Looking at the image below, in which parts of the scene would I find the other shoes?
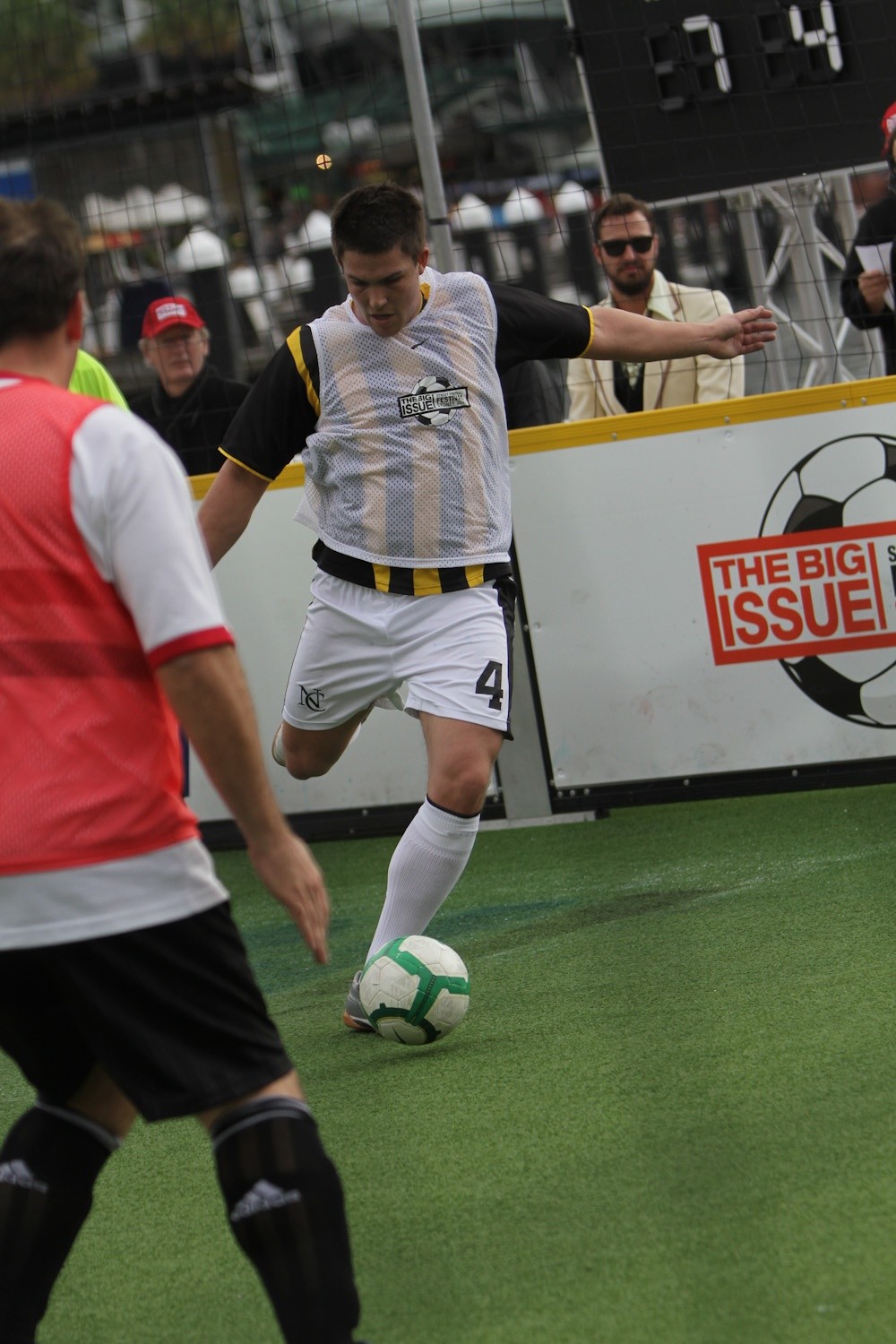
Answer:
[272,723,287,767]
[344,971,376,1031]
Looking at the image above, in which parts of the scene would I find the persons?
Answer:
[0,193,365,1343]
[839,100,896,381]
[562,194,744,424]
[199,186,782,1030]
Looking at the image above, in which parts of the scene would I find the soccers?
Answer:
[358,934,470,1046]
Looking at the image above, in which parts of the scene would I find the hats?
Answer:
[142,297,204,338]
[880,102,896,154]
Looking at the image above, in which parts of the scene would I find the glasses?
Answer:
[602,236,654,257]
